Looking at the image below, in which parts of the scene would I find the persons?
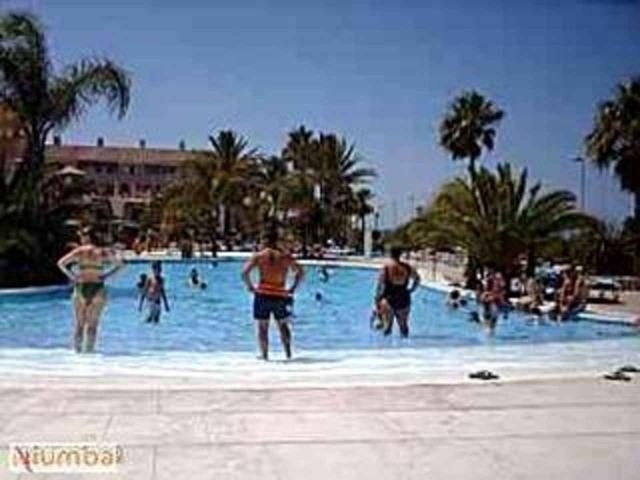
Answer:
[371,245,420,337]
[137,260,170,323]
[312,265,328,304]
[56,224,121,352]
[188,260,218,292]
[240,228,303,360]
[425,244,438,281]
[445,245,591,334]
